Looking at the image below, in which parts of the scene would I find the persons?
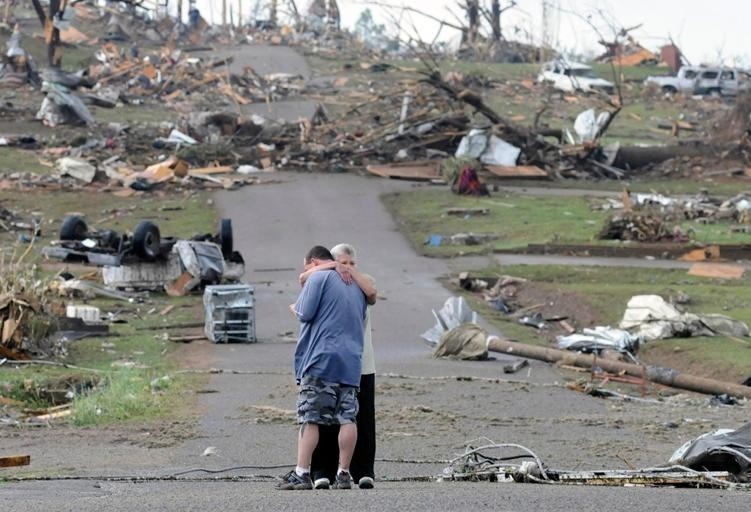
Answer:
[274,243,378,489]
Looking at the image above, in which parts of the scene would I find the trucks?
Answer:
[44,217,246,296]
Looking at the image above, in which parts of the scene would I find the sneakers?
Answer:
[274,470,375,490]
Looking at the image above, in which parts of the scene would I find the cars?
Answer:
[643,65,751,97]
[537,60,614,97]
